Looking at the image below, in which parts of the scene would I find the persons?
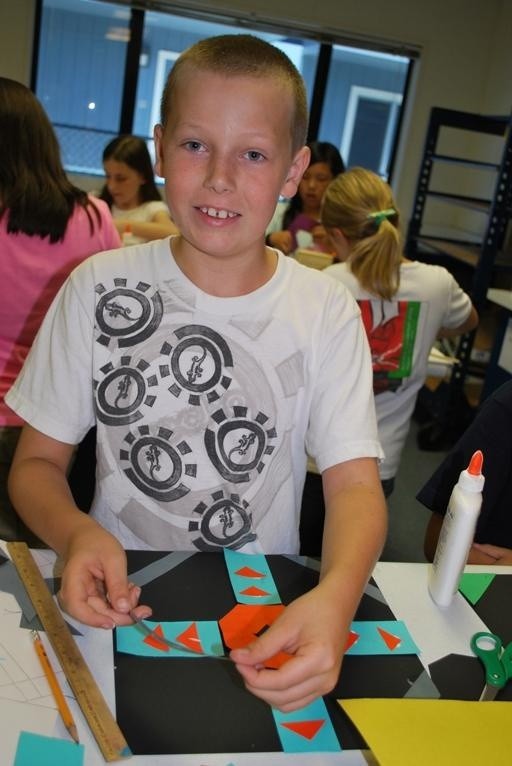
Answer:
[89,133,183,248]
[0,76,124,551]
[262,138,351,266]
[415,378,511,568]
[299,164,481,557]
[2,33,390,716]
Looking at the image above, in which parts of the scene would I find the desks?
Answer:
[0,540,512,766]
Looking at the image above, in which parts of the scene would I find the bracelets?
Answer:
[263,233,274,248]
[124,218,133,234]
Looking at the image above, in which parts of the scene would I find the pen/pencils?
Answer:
[31,628,79,745]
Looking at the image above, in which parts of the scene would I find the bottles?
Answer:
[430,448,487,608]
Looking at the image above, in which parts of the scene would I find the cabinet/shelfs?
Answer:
[398,106,512,451]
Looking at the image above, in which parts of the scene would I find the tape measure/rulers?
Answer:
[7,541,132,763]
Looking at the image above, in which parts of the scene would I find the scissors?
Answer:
[471,632,511,701]
